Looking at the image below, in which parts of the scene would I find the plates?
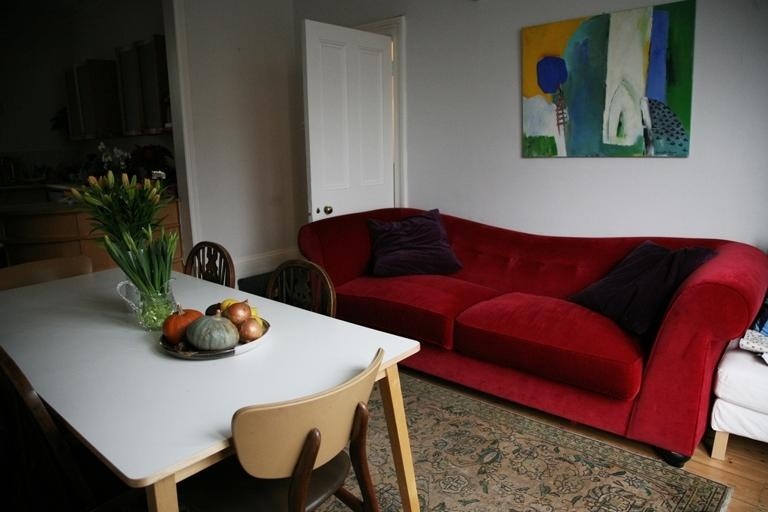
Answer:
[157,318,271,361]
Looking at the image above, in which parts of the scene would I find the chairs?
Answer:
[263,254,336,319]
[178,238,241,290]
[229,344,387,511]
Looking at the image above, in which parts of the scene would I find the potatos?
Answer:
[229,300,253,322]
[242,319,263,341]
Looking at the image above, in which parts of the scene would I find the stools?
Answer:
[705,334,768,463]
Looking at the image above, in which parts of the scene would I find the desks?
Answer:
[0,260,427,511]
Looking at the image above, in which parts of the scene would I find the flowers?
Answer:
[53,164,176,242]
[102,225,184,296]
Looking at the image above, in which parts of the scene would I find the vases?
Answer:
[131,282,187,330]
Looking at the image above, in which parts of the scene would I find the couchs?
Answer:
[294,200,768,468]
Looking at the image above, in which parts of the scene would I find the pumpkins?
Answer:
[159,312,202,345]
[183,308,242,353]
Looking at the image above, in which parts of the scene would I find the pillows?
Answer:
[564,238,718,360]
[362,205,465,281]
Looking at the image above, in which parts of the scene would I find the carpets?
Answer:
[301,358,735,511]
